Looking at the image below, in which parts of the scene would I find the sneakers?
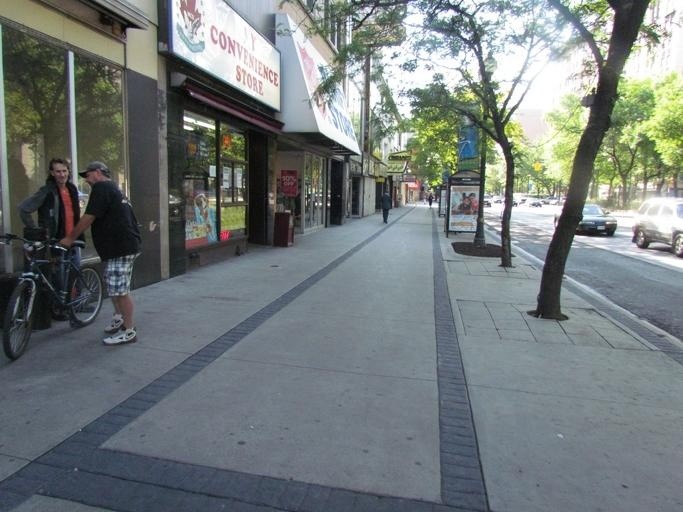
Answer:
[72,301,95,313]
[52,311,69,322]
[101,327,137,347]
[104,312,123,333]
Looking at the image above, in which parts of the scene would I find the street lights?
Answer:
[471,50,498,250]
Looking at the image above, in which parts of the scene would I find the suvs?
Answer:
[627,195,682,257]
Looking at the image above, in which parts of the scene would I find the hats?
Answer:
[78,161,110,179]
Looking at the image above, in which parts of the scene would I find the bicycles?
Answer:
[0,231,103,361]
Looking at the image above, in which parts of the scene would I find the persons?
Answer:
[380,192,391,225]
[17,157,97,322]
[427,192,434,209]
[57,159,145,346]
[452,192,478,215]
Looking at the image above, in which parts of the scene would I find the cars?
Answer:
[481,191,568,210]
[169,193,183,220]
[552,203,617,237]
[72,189,91,210]
